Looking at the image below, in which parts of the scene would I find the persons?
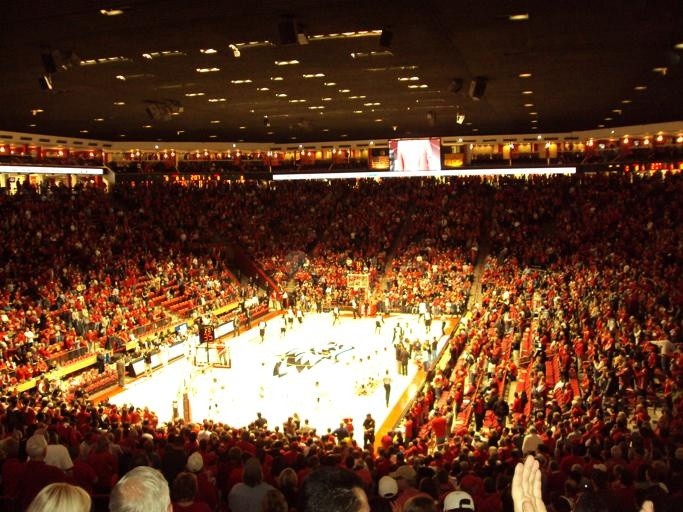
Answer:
[1,153,683,510]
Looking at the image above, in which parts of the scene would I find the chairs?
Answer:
[131,271,193,317]
[452,335,573,436]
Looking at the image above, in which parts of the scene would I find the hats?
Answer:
[279,468,298,487]
[378,476,398,499]
[442,491,474,512]
[593,464,607,472]
[389,466,416,481]
[187,452,203,473]
